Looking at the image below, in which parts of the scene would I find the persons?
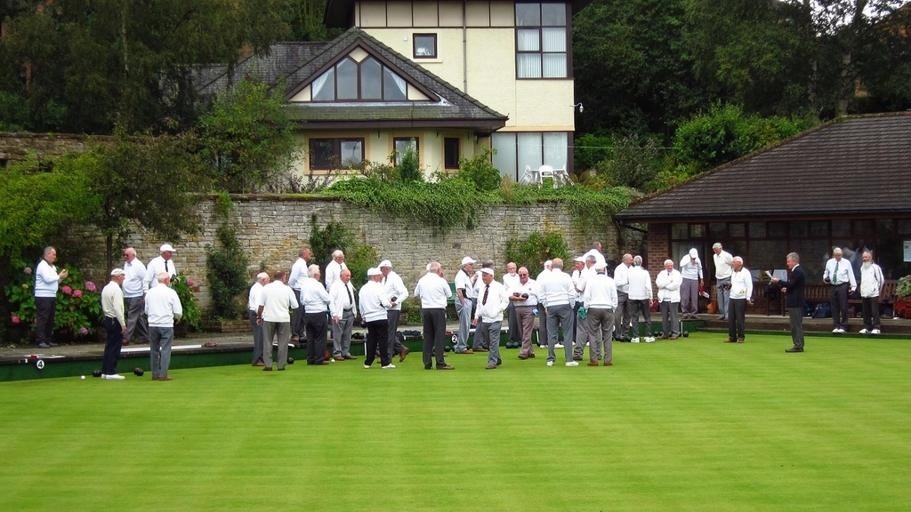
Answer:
[614,254,654,343]
[823,247,857,332]
[656,258,683,340]
[358,260,410,370]
[454,256,618,370]
[712,243,733,321]
[250,271,298,370]
[288,247,357,364]
[583,242,606,266]
[33,245,69,348]
[724,256,754,343]
[769,248,810,355]
[414,261,454,371]
[858,252,887,335]
[679,248,704,320]
[100,242,183,381]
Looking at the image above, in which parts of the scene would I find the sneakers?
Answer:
[832,328,847,334]
[299,337,307,343]
[363,345,409,368]
[102,373,125,380]
[859,328,880,334]
[456,340,612,369]
[736,337,744,343]
[631,333,678,343]
[724,337,737,342]
[291,335,300,342]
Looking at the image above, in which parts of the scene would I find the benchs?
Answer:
[748,279,897,318]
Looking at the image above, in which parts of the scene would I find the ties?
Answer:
[345,284,352,304]
[165,261,168,272]
[833,262,838,283]
[482,284,490,305]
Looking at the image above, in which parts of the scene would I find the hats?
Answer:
[574,257,585,265]
[689,248,699,259]
[461,256,476,265]
[160,244,176,252]
[367,267,382,275]
[379,260,393,268]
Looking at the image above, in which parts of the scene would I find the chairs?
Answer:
[519,165,569,189]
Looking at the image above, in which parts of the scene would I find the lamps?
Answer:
[570,102,583,112]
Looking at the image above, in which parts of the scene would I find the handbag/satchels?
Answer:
[812,303,830,318]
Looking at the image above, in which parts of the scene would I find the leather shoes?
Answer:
[785,347,803,352]
[437,364,453,369]
[691,316,701,319]
[681,316,687,319]
[39,342,57,348]
[307,352,357,364]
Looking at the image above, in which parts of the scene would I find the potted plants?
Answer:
[896,276,911,321]
